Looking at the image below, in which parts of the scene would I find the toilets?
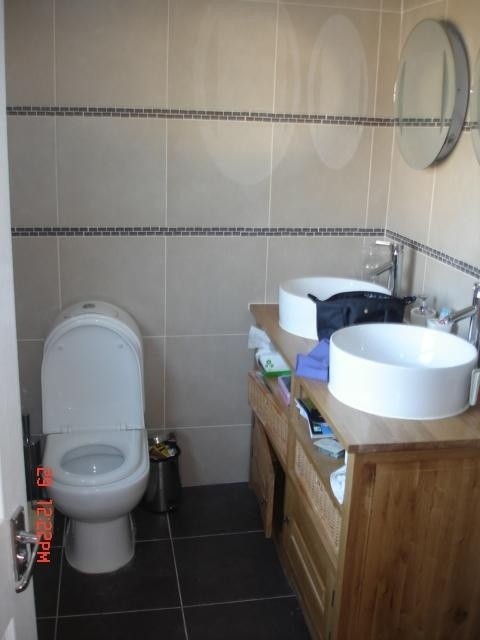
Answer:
[40,299,150,575]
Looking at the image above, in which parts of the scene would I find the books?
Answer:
[294,397,345,459]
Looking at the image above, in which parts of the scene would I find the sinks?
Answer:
[328,322,477,420]
[279,276,392,340]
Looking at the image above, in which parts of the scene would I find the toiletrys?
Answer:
[411,296,435,325]
[427,307,453,332]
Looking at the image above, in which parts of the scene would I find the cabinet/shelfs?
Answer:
[247,303,479,638]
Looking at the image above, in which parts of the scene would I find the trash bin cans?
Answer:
[148,441,182,511]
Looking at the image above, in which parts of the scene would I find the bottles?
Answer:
[364,243,383,285]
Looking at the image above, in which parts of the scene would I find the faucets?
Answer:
[443,280,479,342]
[369,242,398,298]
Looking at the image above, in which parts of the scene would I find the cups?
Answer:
[411,306,453,334]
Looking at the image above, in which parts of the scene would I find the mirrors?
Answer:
[390,18,471,169]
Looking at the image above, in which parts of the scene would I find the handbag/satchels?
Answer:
[308,290,416,342]
